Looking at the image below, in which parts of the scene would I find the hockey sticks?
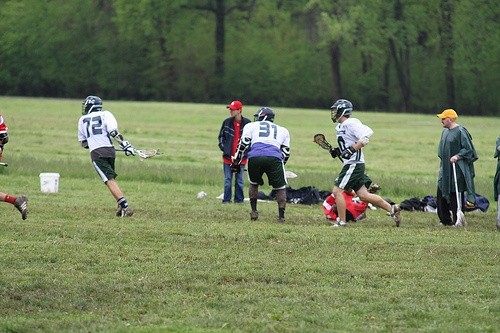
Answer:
[114,149,161,158]
[0,163,8,167]
[452,162,468,228]
[230,167,297,179]
[314,133,343,162]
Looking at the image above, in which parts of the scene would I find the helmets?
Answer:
[82,95,103,115]
[253,107,275,123]
[330,99,352,123]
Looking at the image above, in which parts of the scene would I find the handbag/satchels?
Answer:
[287,185,319,205]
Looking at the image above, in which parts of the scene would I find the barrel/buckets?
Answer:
[39,172,61,193]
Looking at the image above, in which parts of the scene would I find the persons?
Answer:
[230,107,291,222]
[218,100,252,204]
[330,99,401,227]
[77,96,138,219]
[0,116,29,220]
[435,109,478,227]
[494,134,500,231]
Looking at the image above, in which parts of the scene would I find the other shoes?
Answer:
[277,217,285,222]
[251,212,258,220]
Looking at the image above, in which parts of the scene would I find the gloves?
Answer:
[230,157,241,173]
[119,140,136,156]
[342,146,356,160]
[330,147,341,158]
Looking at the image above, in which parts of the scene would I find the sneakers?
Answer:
[387,205,400,227]
[330,217,347,227]
[14,196,29,220]
[121,207,133,217]
[116,205,122,216]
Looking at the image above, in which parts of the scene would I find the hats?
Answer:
[436,109,458,119]
[226,101,242,110]
